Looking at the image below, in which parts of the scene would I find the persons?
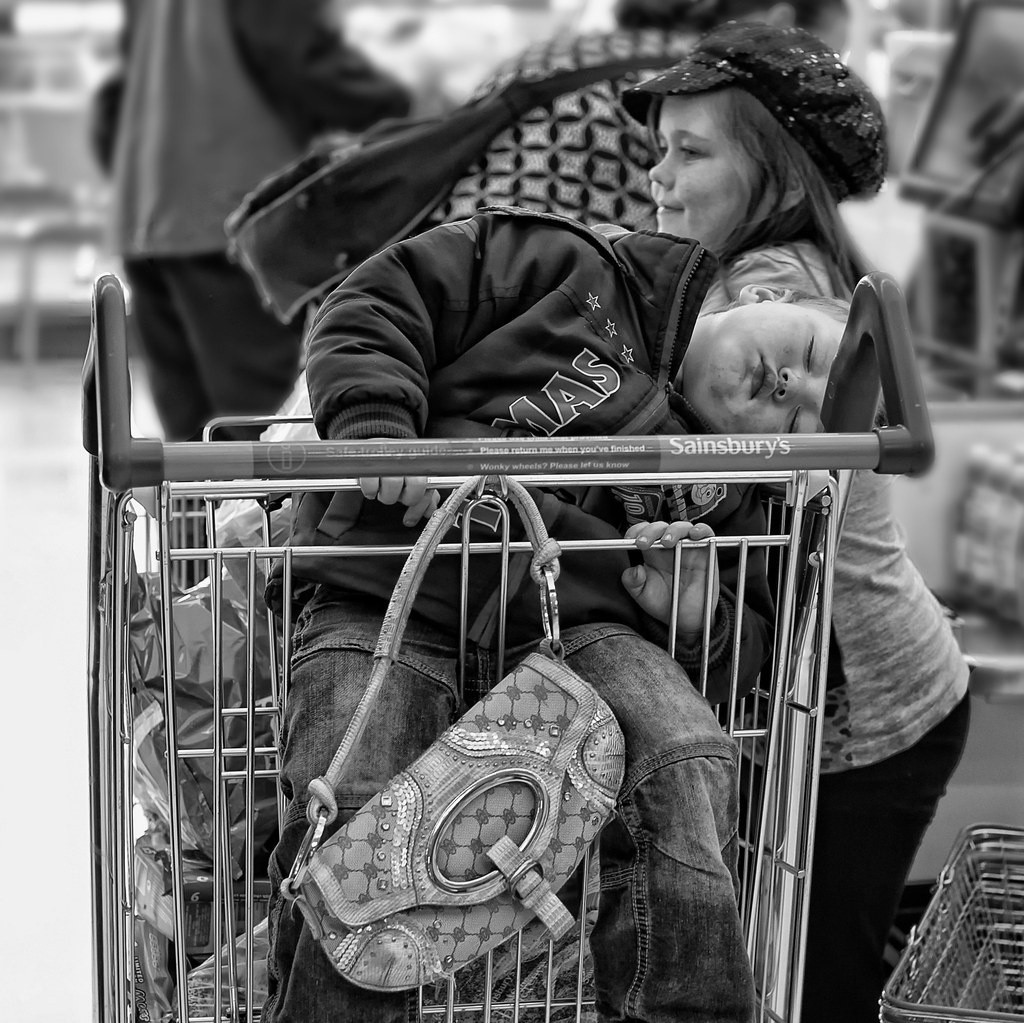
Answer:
[264,0,974,1023]
[88,0,449,583]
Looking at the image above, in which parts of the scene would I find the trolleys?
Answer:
[81,269,936,1022]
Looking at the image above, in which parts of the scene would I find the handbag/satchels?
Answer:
[221,55,684,326]
[279,475,625,992]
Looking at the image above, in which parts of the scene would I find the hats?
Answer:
[620,21,888,203]
